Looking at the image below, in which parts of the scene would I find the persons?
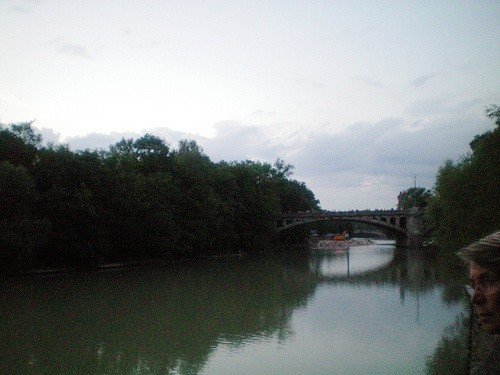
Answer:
[457,232,500,375]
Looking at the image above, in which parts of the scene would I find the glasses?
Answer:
[466,270,500,298]
